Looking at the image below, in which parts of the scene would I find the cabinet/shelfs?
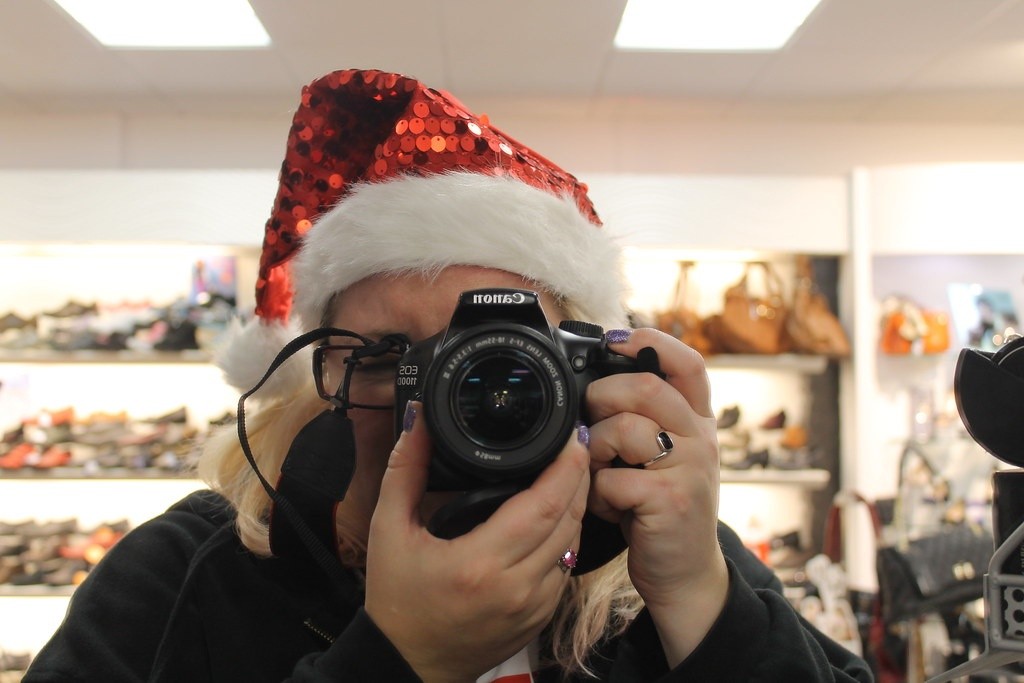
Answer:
[0,349,830,683]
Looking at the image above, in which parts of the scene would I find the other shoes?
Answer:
[714,407,818,569]
[0,292,236,669]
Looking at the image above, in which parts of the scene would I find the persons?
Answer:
[15,67,876,683]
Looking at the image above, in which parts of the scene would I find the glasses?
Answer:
[312,343,401,410]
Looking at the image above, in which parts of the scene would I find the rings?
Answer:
[557,548,578,575]
[639,428,676,469]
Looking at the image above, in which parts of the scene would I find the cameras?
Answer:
[392,287,667,579]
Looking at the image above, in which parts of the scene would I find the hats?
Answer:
[215,67,630,396]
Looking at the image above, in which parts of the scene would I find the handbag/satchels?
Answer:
[875,443,995,624]
[877,300,950,355]
[804,488,878,656]
[658,259,852,358]
